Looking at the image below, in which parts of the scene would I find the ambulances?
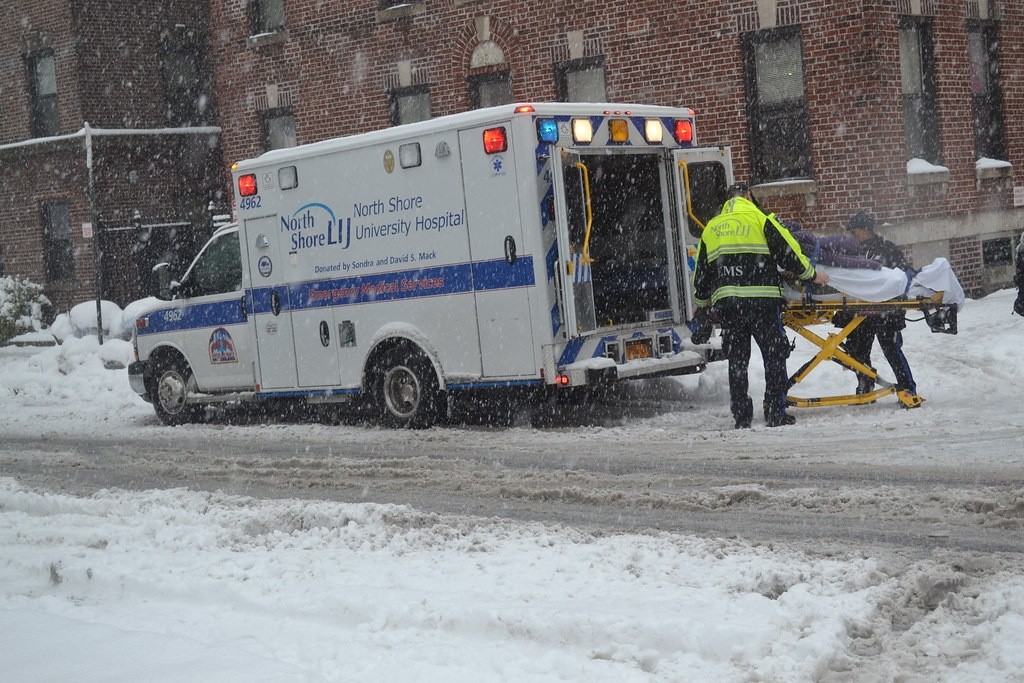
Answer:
[126,101,737,429]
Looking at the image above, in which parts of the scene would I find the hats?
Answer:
[727,183,759,207]
[847,211,873,230]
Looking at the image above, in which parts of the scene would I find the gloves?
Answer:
[694,307,707,325]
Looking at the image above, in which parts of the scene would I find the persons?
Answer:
[846,211,917,405]
[693,183,829,430]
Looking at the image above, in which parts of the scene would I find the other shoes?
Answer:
[901,388,921,409]
[856,384,872,394]
[735,414,752,429]
[766,412,795,427]
